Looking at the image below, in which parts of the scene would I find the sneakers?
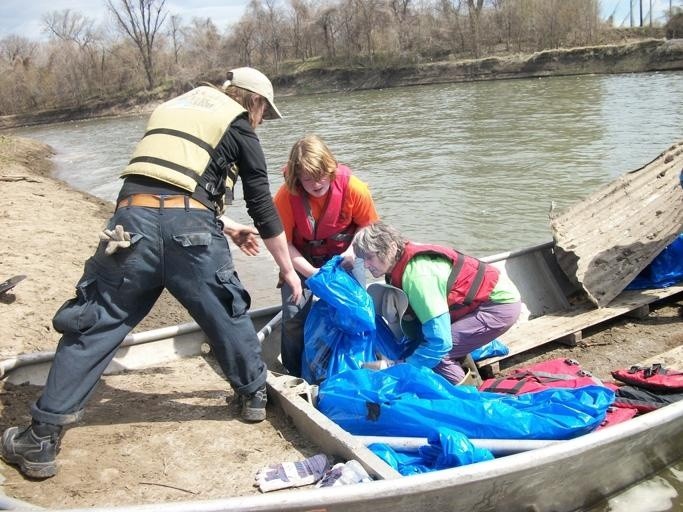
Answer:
[235,381,268,421]
[2,421,64,479]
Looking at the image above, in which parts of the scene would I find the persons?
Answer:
[0,66,301,481]
[272,137,380,378]
[350,223,523,386]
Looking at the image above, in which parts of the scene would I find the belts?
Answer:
[116,194,209,210]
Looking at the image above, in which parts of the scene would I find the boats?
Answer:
[0,144,681,507]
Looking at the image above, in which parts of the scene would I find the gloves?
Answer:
[254,454,329,493]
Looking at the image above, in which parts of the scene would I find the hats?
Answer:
[226,67,282,121]
